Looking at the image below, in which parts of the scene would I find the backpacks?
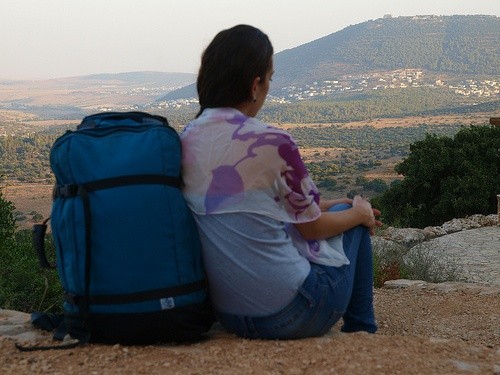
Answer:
[14,112,213,351]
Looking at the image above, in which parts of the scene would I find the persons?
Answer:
[178,25,383,341]
